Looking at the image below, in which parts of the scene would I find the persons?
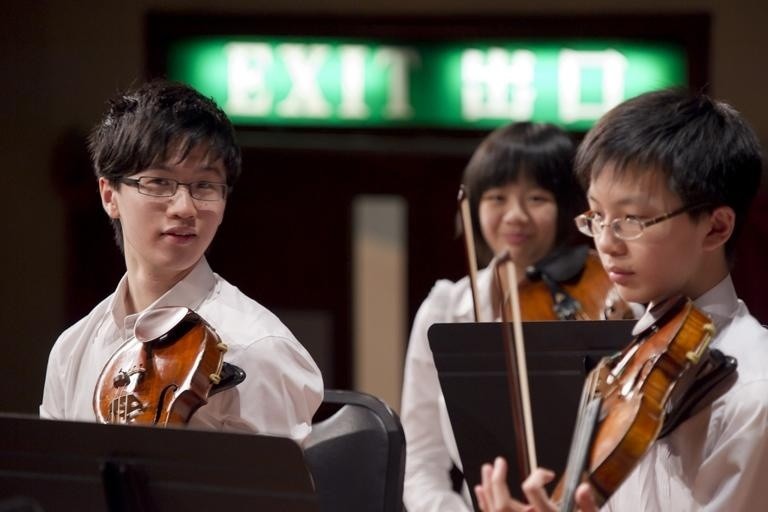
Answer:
[473,89,768,512]
[39,77,323,444]
[401,123,644,512]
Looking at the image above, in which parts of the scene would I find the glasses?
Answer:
[120,174,233,202]
[573,206,690,242]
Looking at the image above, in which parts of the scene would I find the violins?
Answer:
[551,296,739,510]
[505,249,618,323]
[93,309,245,423]
